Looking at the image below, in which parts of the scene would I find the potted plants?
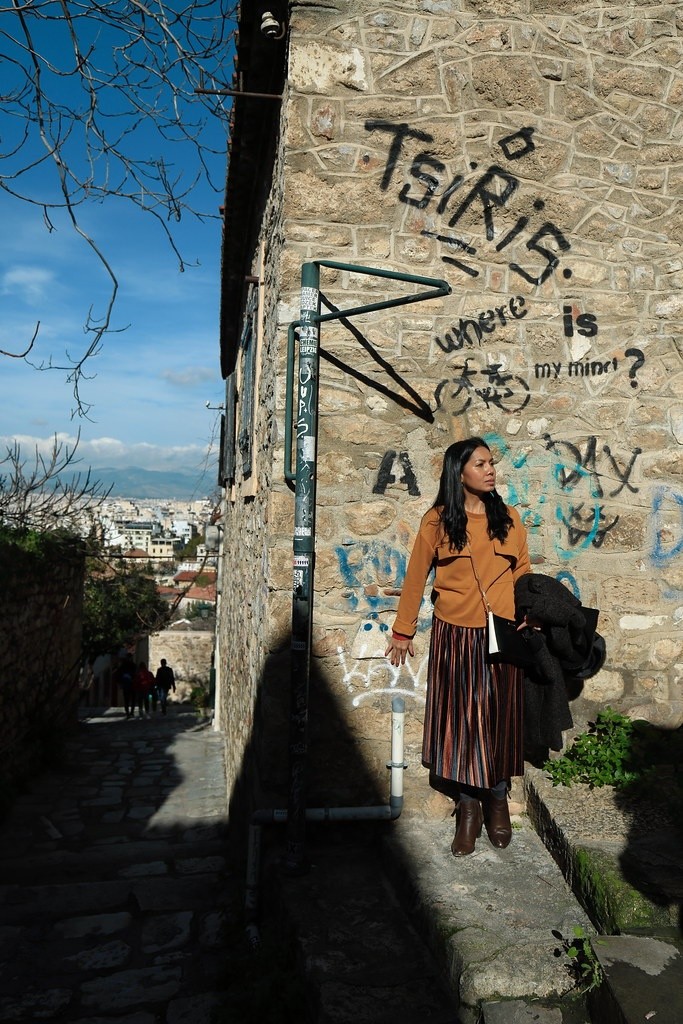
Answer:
[192,695,211,717]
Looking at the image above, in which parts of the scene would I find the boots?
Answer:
[451,790,512,857]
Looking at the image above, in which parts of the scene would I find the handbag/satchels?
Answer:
[487,611,527,667]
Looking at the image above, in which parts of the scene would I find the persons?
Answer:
[384,435,545,858]
[131,662,156,712]
[154,659,176,716]
[116,653,138,718]
